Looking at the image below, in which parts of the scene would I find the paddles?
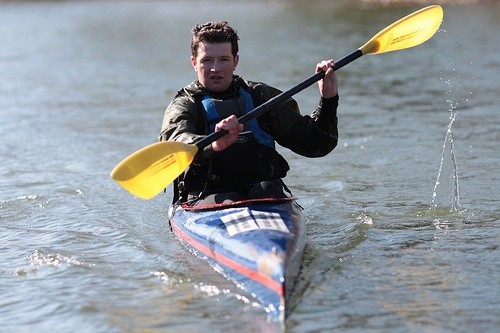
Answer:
[108,4,444,200]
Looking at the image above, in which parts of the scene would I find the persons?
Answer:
[160,20,340,204]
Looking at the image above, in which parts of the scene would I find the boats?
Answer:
[166,196,307,323]
